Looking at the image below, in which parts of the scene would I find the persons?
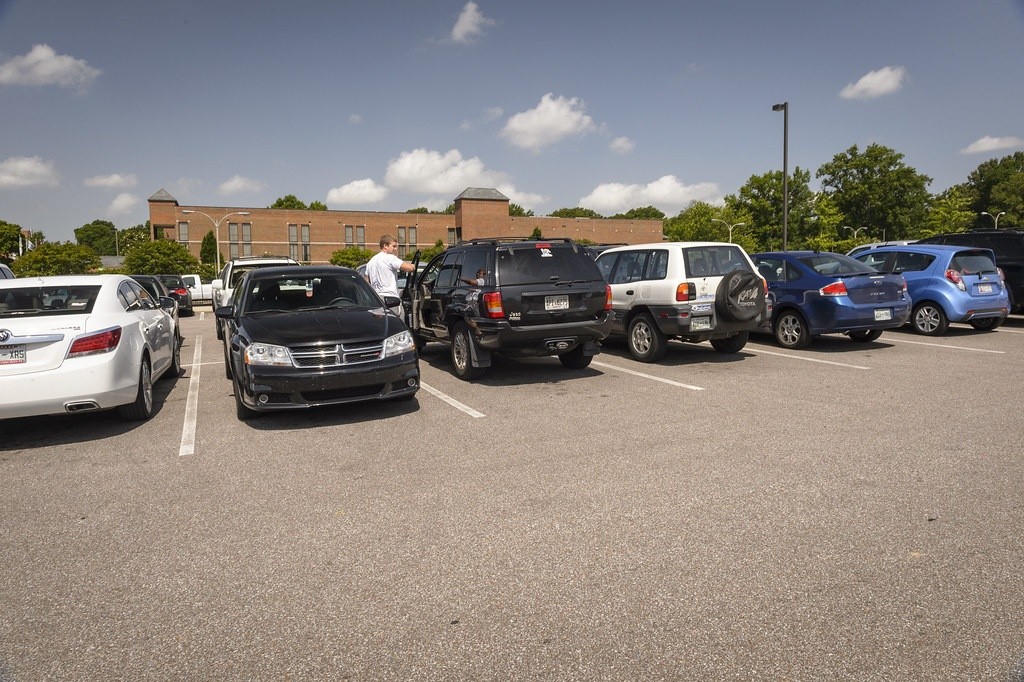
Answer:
[459,269,486,342]
[364,234,414,323]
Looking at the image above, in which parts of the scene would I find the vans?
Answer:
[355,259,439,296]
[846,227,1023,312]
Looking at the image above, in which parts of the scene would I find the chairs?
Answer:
[251,280,288,310]
[311,277,343,307]
[628,262,641,282]
[761,265,778,280]
[694,258,709,275]
[15,295,46,310]
[897,253,913,271]
[65,293,90,309]
[170,281,177,285]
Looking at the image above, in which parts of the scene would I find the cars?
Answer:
[0,262,17,279]
[128,274,179,306]
[216,266,421,424]
[210,255,304,313]
[0,273,186,422]
[154,274,192,316]
[832,245,1011,337]
[720,251,912,350]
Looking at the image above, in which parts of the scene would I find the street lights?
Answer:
[182,210,250,275]
[711,218,745,244]
[772,101,789,256]
[979,210,1007,230]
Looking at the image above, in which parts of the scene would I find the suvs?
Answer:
[400,236,617,383]
[595,242,768,365]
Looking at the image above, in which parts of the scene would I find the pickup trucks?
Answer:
[176,274,213,301]
